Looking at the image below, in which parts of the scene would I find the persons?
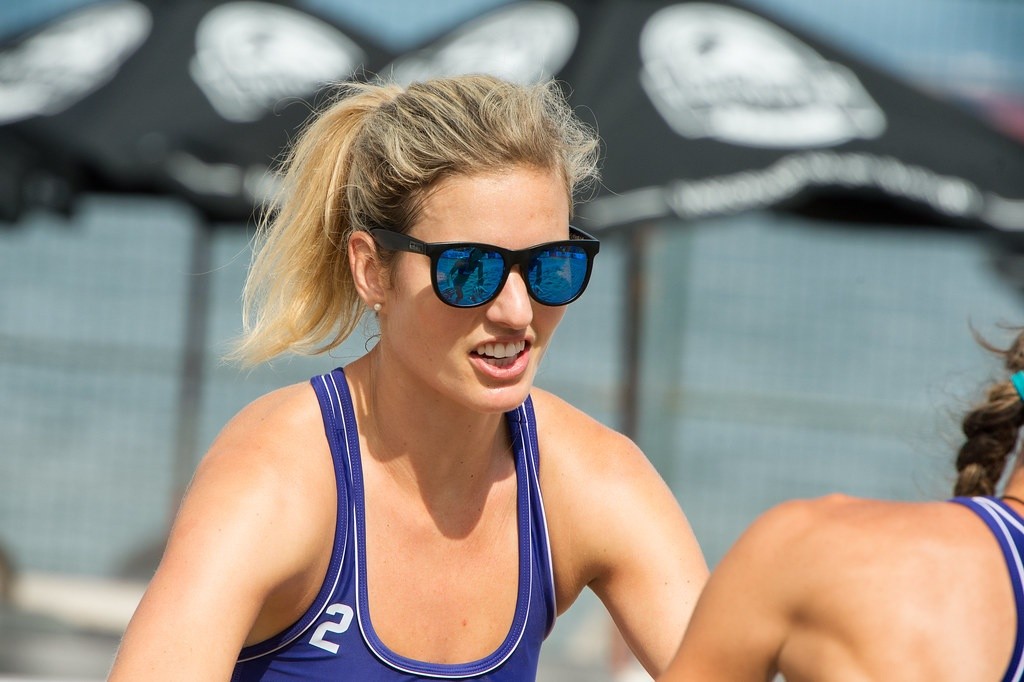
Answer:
[657,330,1023,681]
[105,76,712,682]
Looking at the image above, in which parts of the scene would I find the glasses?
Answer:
[370,221,601,309]
